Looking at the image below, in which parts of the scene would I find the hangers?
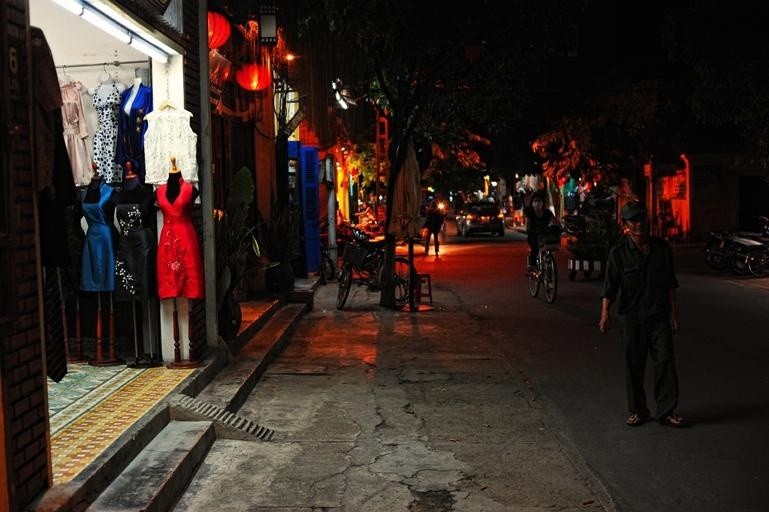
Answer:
[142,96,194,120]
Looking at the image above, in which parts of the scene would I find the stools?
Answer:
[406,275,433,307]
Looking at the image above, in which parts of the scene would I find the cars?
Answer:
[456,201,506,239]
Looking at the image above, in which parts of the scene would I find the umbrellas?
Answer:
[386,142,421,310]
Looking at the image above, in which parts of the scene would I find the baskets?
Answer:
[341,245,367,265]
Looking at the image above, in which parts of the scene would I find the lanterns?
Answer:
[235,62,272,91]
[208,12,232,51]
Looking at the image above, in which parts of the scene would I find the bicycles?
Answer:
[321,224,421,310]
[525,228,562,303]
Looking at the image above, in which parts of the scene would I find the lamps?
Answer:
[52,0,171,64]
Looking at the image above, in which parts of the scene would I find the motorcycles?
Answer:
[703,217,768,278]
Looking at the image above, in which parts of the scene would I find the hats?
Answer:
[620,201,646,218]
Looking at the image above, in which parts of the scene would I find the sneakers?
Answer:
[626,414,646,426]
[659,415,682,426]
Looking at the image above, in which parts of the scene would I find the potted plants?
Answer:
[217,168,254,339]
[265,211,302,293]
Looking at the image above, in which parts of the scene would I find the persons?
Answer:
[114,77,151,186]
[154,170,201,302]
[523,190,561,278]
[80,175,121,291]
[58,74,96,187]
[107,173,160,302]
[598,201,689,429]
[87,73,130,185]
[421,201,445,257]
